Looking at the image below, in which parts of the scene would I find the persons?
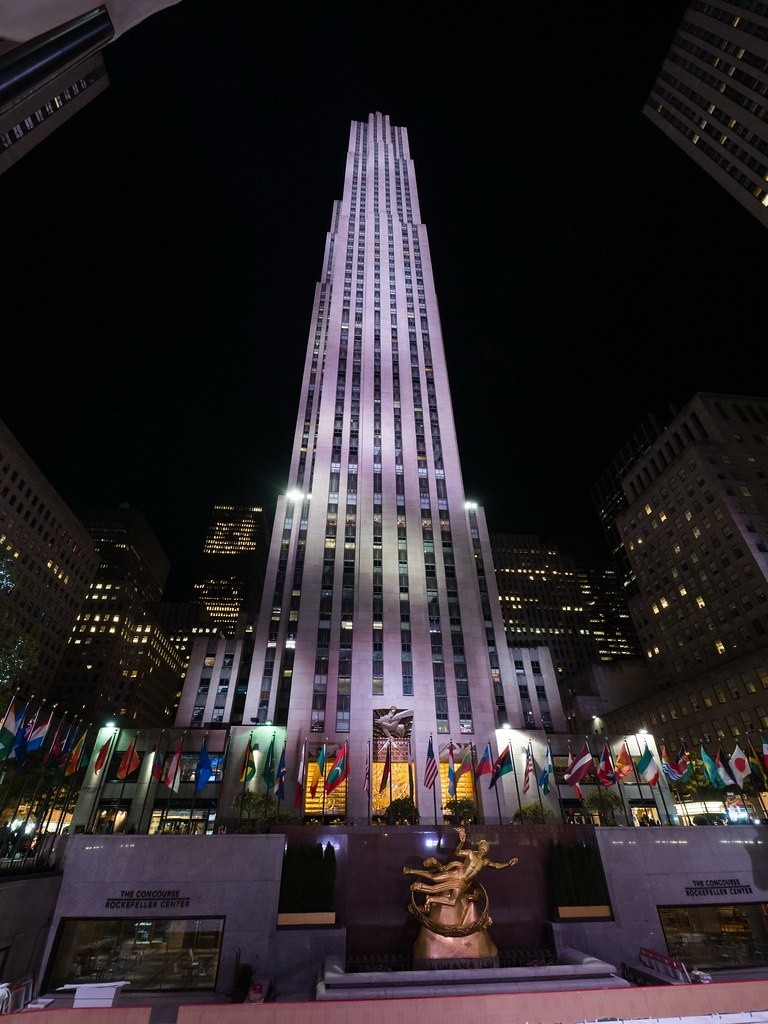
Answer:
[404,826,521,913]
[639,816,755,827]
[0,819,226,861]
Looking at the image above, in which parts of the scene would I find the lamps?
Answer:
[253,744,259,750]
[251,717,259,725]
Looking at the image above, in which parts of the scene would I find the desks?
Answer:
[90,948,220,988]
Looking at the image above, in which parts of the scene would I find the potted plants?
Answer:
[548,838,611,917]
[277,840,336,923]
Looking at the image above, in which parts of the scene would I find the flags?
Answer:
[0,688,767,808]
[327,739,350,794]
